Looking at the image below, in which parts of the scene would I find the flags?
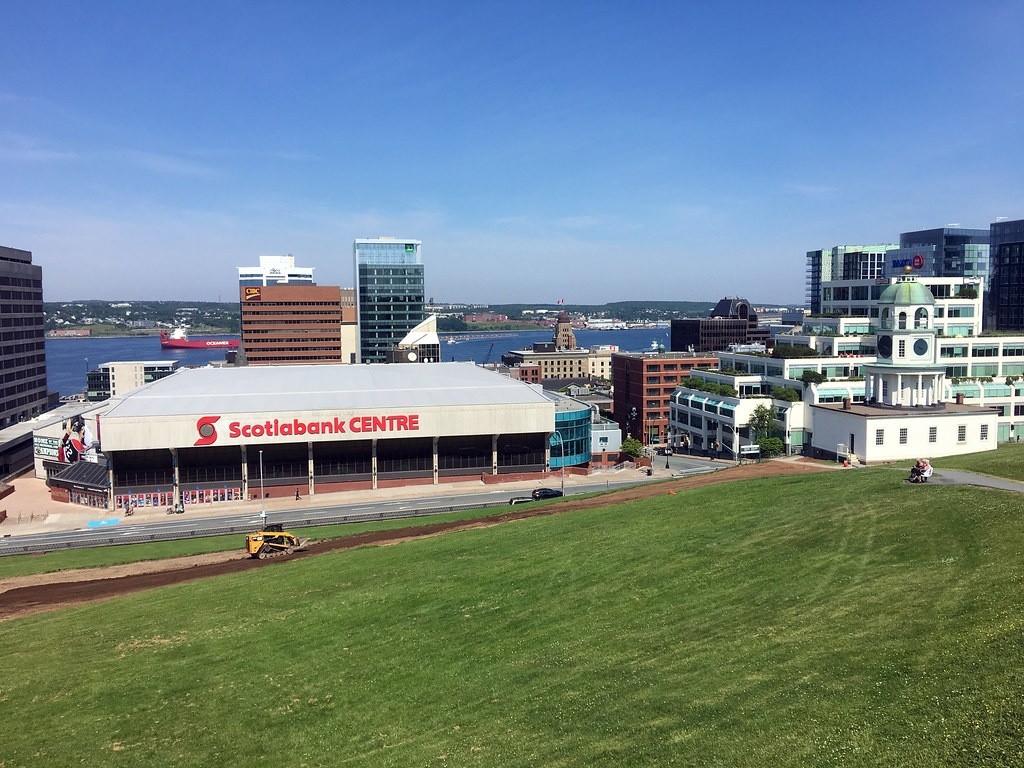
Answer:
[558,299,563,304]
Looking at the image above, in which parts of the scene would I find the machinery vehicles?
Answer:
[245,509,312,560]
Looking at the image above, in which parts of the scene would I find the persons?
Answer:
[57,415,94,463]
[909,458,929,482]
[124,501,184,517]
[295,488,301,501]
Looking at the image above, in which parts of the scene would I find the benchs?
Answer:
[921,465,934,482]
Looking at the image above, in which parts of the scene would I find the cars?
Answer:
[509,497,534,505]
[531,488,563,500]
[657,447,673,457]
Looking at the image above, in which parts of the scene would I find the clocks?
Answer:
[914,339,928,355]
[878,335,892,359]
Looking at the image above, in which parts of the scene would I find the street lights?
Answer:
[553,431,565,499]
[259,450,268,528]
[652,416,662,450]
[724,424,738,467]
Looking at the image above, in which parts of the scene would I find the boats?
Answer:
[159,328,240,348]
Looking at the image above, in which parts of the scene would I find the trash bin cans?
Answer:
[248,494,252,501]
[711,455,714,460]
[647,469,652,476]
